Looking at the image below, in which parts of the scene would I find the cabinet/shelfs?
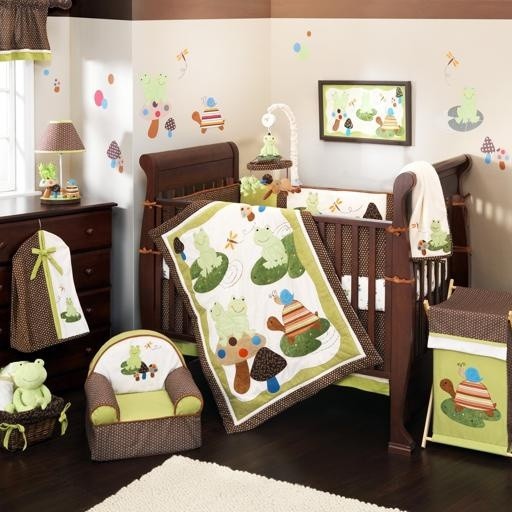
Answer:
[3,190,121,394]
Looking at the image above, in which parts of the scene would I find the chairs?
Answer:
[82,327,204,463]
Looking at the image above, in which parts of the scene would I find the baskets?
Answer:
[0,394,71,453]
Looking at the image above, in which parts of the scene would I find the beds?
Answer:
[137,137,473,454]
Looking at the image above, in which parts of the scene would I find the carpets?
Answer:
[85,454,407,512]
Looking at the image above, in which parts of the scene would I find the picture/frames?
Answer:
[317,80,413,147]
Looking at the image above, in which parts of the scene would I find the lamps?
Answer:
[36,121,86,207]
